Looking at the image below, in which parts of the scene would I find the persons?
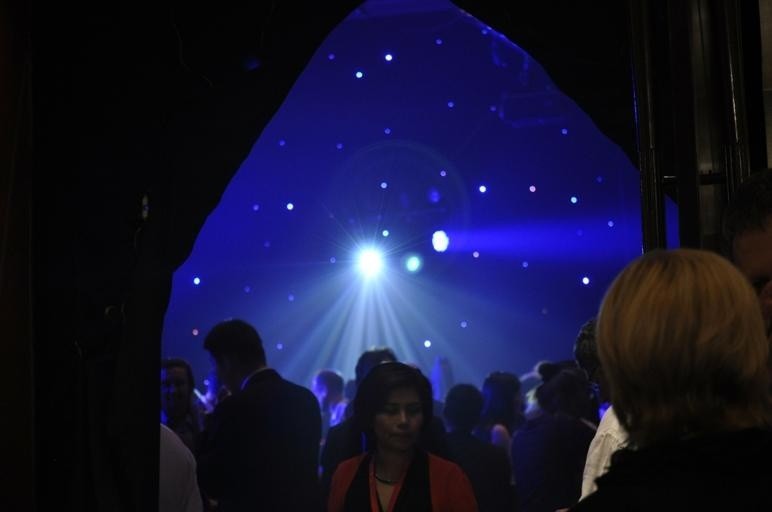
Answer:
[161,424,202,512]
[578,247,772,512]
[161,358,204,448]
[439,383,513,512]
[508,363,599,512]
[574,316,630,503]
[324,345,445,486]
[314,370,346,428]
[481,370,525,457]
[326,361,479,512]
[200,317,326,512]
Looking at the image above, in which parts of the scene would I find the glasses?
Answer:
[588,364,600,384]
